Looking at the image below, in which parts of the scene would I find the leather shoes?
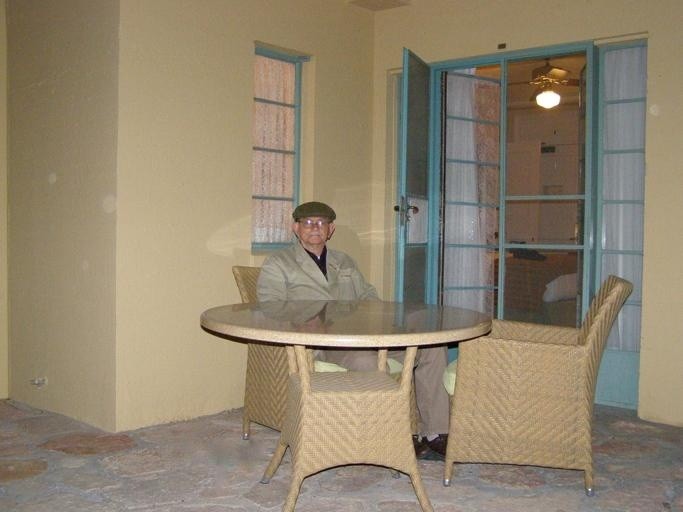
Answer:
[426,434,446,455]
[413,435,432,458]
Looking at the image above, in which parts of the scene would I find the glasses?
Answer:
[299,219,328,227]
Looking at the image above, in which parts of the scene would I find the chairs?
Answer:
[441,272,634,498]
[230,264,419,440]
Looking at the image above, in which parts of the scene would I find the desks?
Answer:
[199,298,492,512]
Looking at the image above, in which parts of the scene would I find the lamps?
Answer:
[528,76,569,110]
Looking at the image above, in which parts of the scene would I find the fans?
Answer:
[508,58,586,101]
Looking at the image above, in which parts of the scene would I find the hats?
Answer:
[293,201,336,221]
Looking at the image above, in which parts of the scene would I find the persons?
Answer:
[253,200,451,459]
